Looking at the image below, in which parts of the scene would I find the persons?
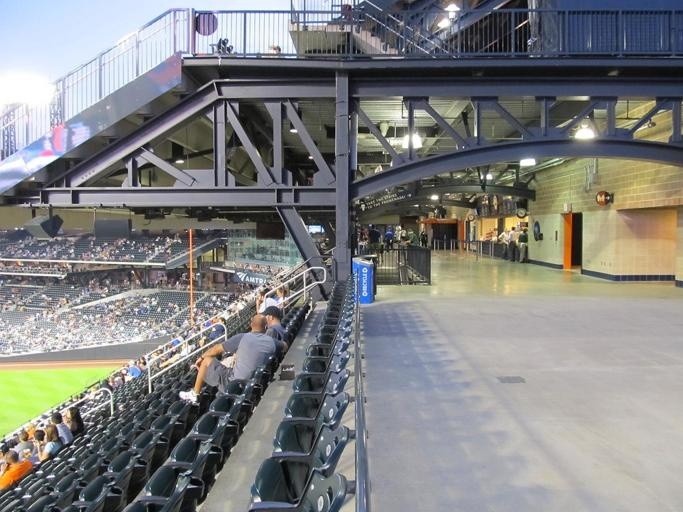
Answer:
[0,235,63,356]
[497,228,508,259]
[359,221,428,266]
[0,406,84,491]
[505,226,516,261]
[517,226,529,262]
[63,234,296,407]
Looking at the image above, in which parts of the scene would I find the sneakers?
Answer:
[178,390,198,402]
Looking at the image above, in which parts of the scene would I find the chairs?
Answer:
[0,276,317,509]
[247,271,356,511]
[1,233,227,276]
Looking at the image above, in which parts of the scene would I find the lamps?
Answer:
[573,115,596,140]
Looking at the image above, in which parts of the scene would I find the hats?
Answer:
[260,306,279,317]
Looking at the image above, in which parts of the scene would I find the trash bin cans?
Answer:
[362,254,378,295]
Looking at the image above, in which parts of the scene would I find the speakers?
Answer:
[20,216,61,239]
